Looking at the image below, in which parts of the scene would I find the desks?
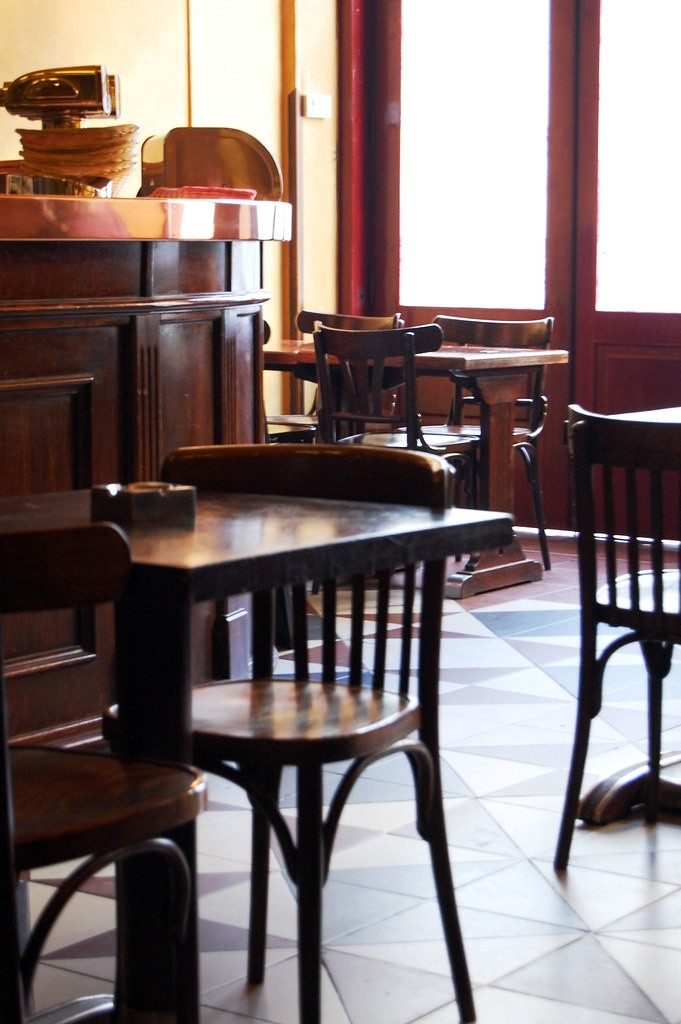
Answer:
[0,476,516,1023]
[259,338,572,602]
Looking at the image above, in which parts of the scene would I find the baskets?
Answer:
[15,123,140,197]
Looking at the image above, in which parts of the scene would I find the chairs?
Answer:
[547,403,681,869]
[295,310,553,571]
[99,440,478,1024]
[0,637,208,1023]
